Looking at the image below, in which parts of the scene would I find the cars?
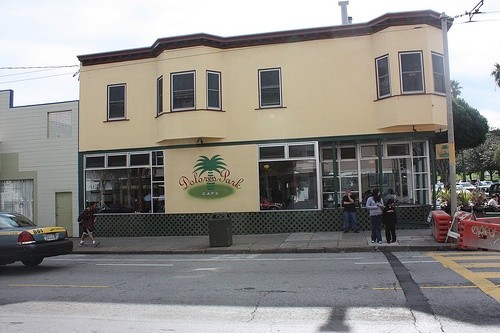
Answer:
[434,182,446,191]
[259,198,282,209]
[0,213,73,268]
[455,180,500,197]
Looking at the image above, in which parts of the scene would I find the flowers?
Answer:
[469,186,488,208]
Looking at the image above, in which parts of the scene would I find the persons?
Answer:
[383,199,397,243]
[488,194,500,208]
[383,189,396,202]
[343,189,360,233]
[364,190,372,202]
[366,189,384,244]
[78,202,100,248]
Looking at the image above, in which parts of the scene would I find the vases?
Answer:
[473,207,485,218]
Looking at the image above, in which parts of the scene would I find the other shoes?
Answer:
[391,240,395,242]
[79,243,87,246]
[344,231,347,233]
[354,231,359,233]
[379,241,382,243]
[387,240,390,243]
[372,240,374,242]
[94,242,100,247]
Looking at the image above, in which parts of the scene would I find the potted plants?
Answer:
[457,189,472,213]
[442,193,451,215]
